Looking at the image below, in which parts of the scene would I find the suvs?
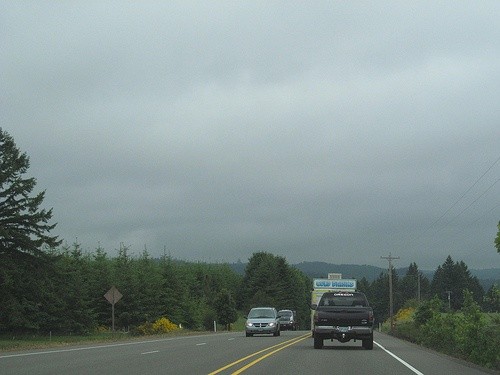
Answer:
[310,290,375,350]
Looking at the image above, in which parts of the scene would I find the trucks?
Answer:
[278,309,297,331]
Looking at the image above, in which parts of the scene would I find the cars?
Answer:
[244,306,281,336]
